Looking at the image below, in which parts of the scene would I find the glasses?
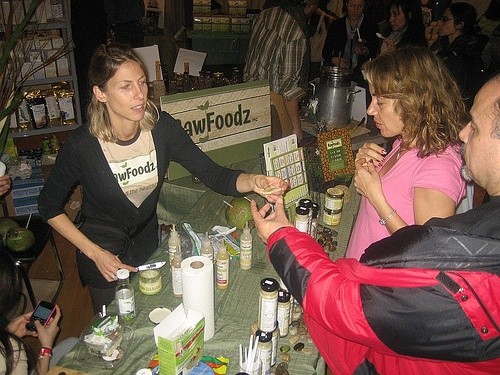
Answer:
[441,14,459,23]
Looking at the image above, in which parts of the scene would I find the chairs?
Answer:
[0,194,65,309]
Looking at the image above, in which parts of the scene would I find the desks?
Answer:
[190,31,251,66]
[297,102,393,152]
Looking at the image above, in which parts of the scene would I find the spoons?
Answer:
[356,28,362,42]
[376,32,385,39]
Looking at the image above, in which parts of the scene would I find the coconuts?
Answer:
[226,196,257,229]
[0,218,36,251]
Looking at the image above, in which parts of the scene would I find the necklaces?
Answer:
[397,153,399,159]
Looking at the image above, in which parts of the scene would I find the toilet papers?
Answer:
[181,256,215,341]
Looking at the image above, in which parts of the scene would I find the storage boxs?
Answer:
[10,168,44,216]
[192,0,260,32]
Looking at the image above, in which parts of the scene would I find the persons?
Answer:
[424,3,486,83]
[422,0,438,21]
[318,0,344,29]
[104,0,145,47]
[378,0,426,54]
[251,75,500,375]
[322,0,379,69]
[346,47,469,261]
[36,43,290,320]
[243,0,319,144]
[0,243,79,375]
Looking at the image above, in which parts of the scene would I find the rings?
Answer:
[112,275,114,279]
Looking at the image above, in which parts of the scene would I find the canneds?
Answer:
[294,200,319,239]
[41,137,60,152]
[139,269,162,295]
[255,278,303,375]
[323,187,344,225]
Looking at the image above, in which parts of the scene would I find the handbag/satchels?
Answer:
[309,13,326,62]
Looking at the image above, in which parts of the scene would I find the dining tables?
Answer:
[48,158,362,375]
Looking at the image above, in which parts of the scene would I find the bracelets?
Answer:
[40,348,52,357]
[379,210,396,225]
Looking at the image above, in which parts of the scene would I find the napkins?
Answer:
[153,303,205,375]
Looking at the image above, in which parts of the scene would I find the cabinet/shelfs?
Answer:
[0,0,82,138]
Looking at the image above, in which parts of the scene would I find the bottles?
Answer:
[171,245,183,296]
[169,224,181,267]
[200,231,214,263]
[114,268,136,323]
[168,62,240,95]
[240,222,252,270]
[216,239,229,289]
[153,61,166,103]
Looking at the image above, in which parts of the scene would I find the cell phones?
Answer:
[26,301,55,333]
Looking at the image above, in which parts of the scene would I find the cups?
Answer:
[238,358,262,375]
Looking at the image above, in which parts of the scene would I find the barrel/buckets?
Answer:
[318,64,352,130]
